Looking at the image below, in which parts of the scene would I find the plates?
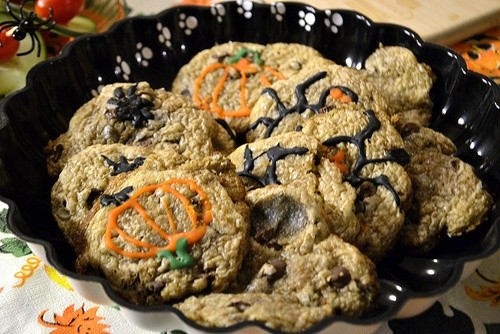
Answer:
[1,0,499,334]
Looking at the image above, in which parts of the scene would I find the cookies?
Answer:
[42,44,489,327]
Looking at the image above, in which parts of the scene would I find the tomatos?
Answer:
[0,24,21,66]
[36,1,85,24]
